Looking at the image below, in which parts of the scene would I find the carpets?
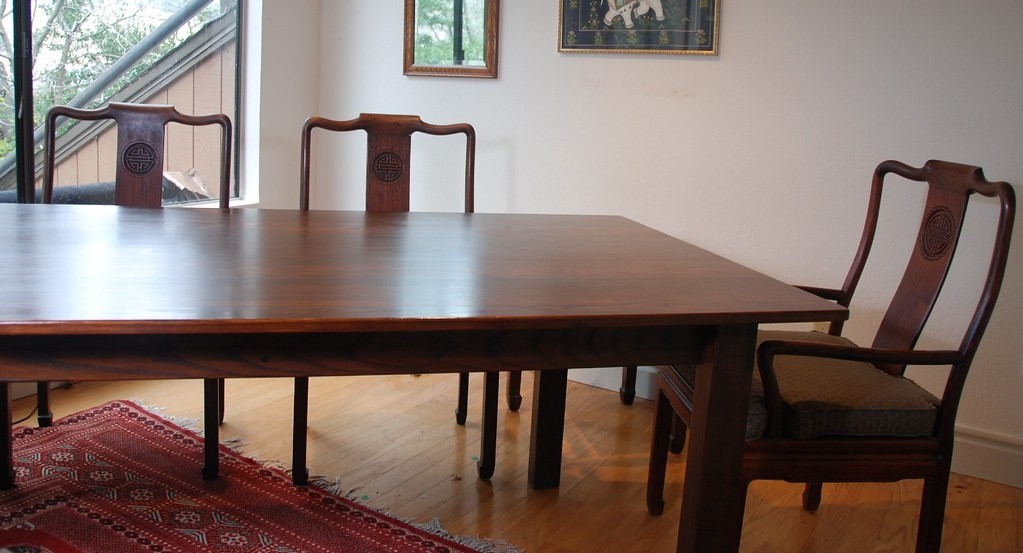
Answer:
[0,396,525,553]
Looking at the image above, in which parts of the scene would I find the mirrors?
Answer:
[403,0,500,79]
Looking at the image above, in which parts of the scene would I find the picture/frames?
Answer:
[556,0,720,55]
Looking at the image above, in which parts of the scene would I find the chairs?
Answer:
[0,107,233,489]
[648,158,1018,553]
[292,113,500,486]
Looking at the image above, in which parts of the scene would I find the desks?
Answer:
[0,204,850,553]
